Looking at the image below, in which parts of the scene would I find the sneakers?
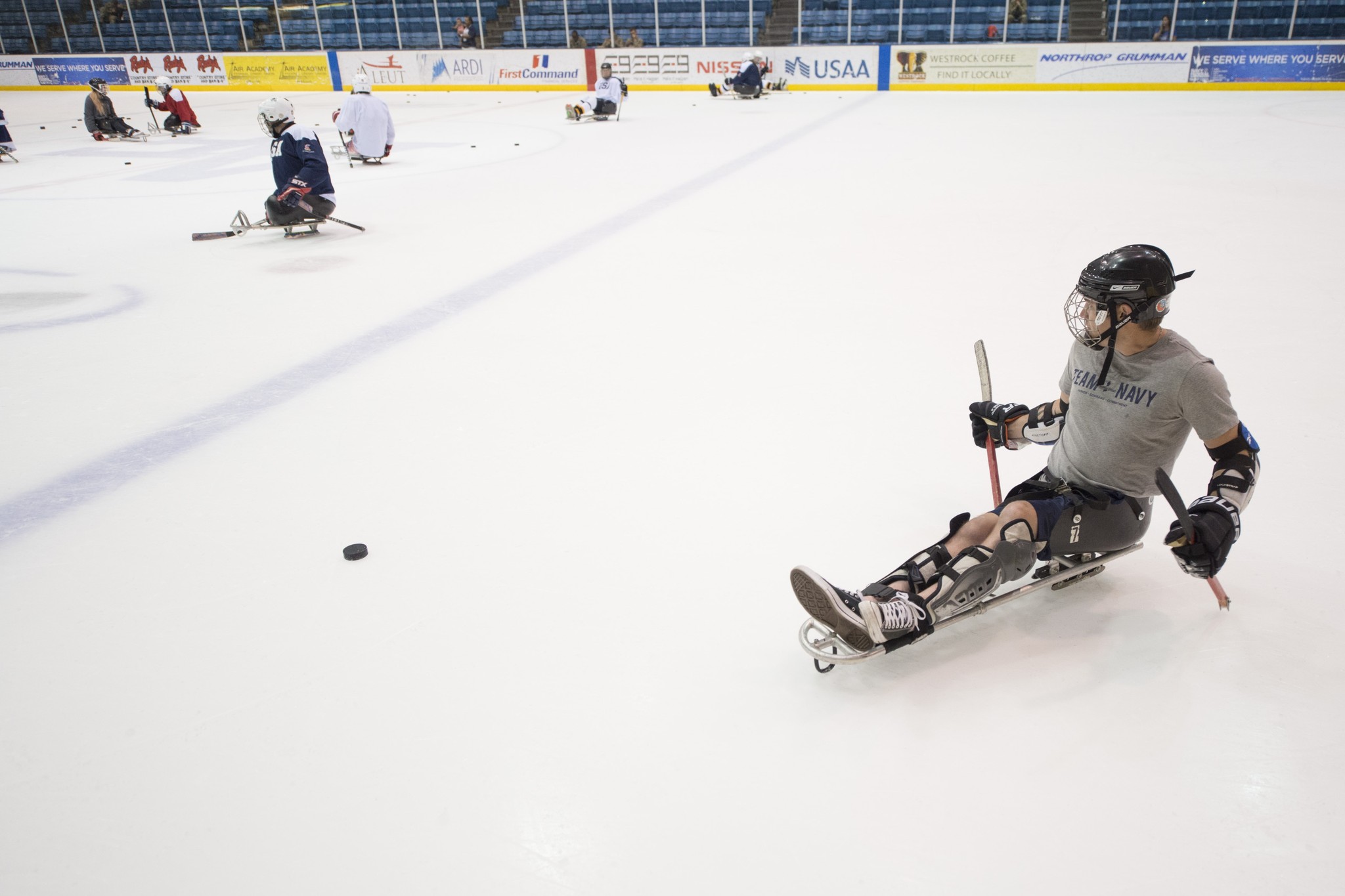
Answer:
[565,105,584,122]
[709,83,718,97]
[791,566,874,653]
[773,77,787,90]
[858,595,924,644]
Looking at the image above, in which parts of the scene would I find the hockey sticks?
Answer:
[338,129,353,168]
[617,77,625,121]
[720,64,736,100]
[298,199,365,232]
[764,56,768,79]
[144,86,161,133]
[974,339,1002,509]
[0,145,19,163]
[192,219,267,241]
[91,135,121,142]
[1154,466,1231,611]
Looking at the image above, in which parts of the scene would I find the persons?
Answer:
[601,28,642,48]
[144,76,201,128]
[1008,0,1028,24]
[258,96,335,223]
[566,63,627,117]
[334,74,395,160]
[1153,15,1177,41]
[452,15,477,50]
[84,78,144,141]
[797,245,1260,649]
[100,0,123,24]
[710,51,787,97]
[570,30,587,49]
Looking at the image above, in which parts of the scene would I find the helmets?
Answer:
[156,76,171,95]
[600,62,612,81]
[89,76,106,99]
[741,50,763,68]
[1063,245,1177,350]
[352,74,370,93]
[258,97,294,137]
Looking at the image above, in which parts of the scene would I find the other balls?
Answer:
[342,543,368,561]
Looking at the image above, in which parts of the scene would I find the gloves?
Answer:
[384,141,393,157]
[969,403,1032,450]
[92,131,104,141]
[1165,496,1240,577]
[183,123,191,134]
[760,67,769,75]
[277,178,307,209]
[724,78,734,86]
[621,84,628,97]
[145,98,158,110]
[332,110,340,122]
